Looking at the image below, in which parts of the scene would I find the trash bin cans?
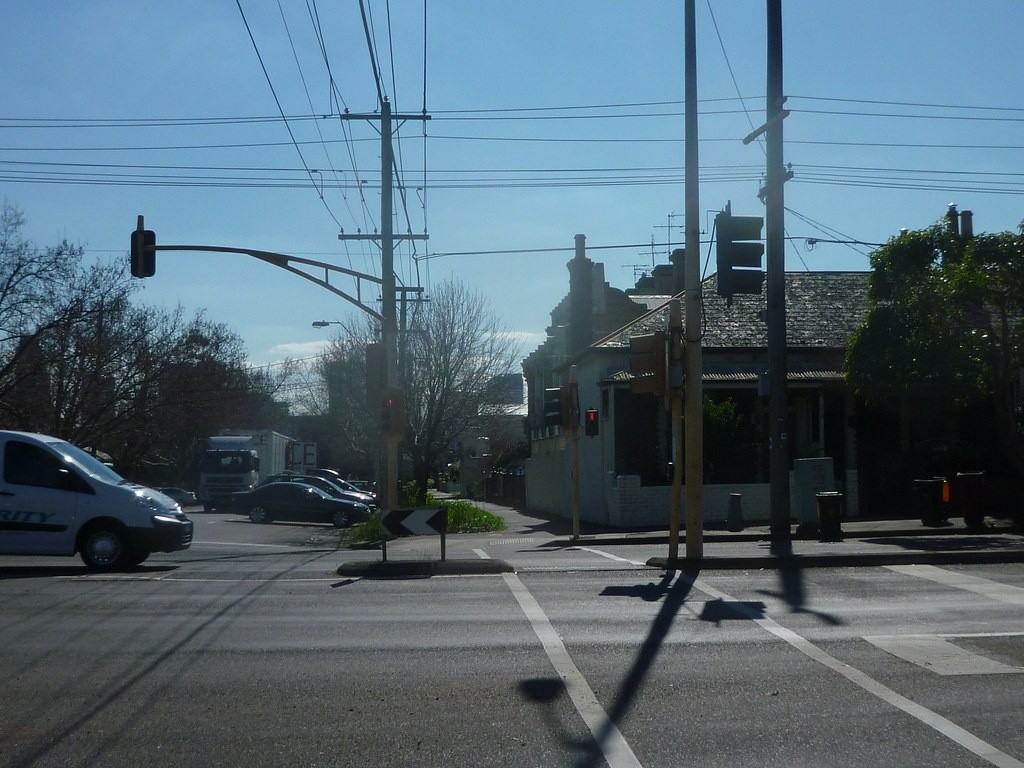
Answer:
[956,471,984,525]
[914,476,951,527]
[816,491,844,542]
[727,493,744,532]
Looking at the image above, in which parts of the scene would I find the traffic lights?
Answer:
[715,199,766,297]
[587,409,599,438]
[378,389,399,439]
[544,386,566,428]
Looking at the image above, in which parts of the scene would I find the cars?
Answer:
[232,467,376,528]
[151,487,196,508]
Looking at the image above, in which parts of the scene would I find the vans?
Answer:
[0,430,194,571]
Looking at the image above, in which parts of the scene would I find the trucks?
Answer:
[199,428,317,512]
